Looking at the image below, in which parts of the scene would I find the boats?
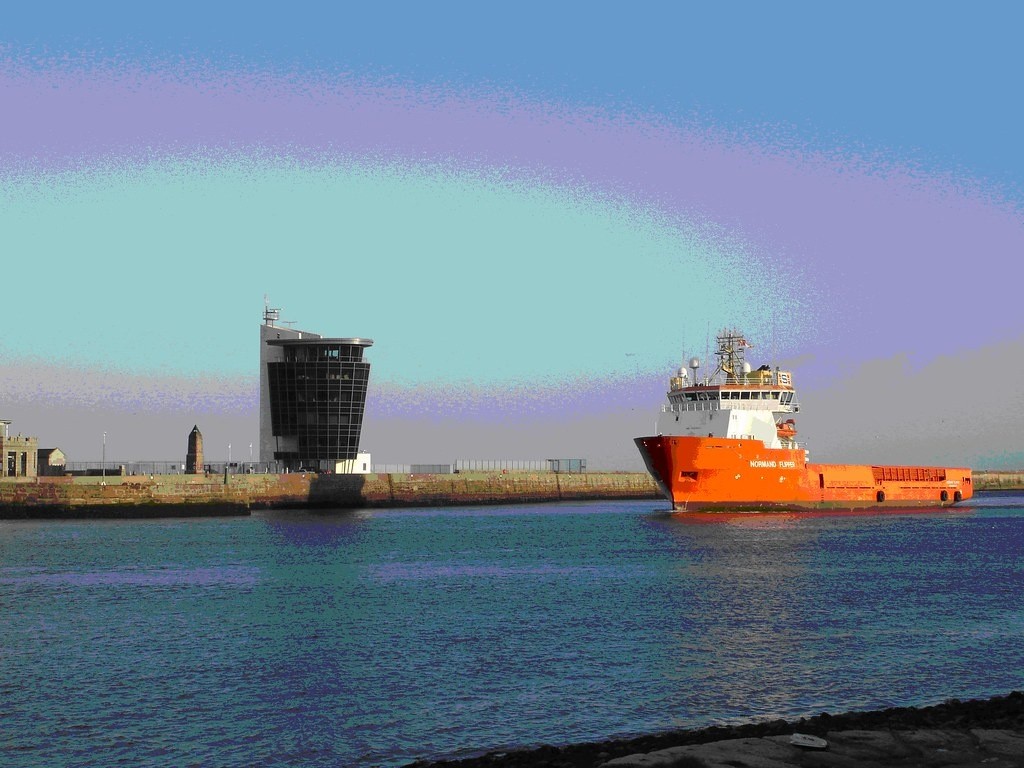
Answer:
[776,419,798,438]
[632,321,974,512]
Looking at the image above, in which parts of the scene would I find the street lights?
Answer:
[249,443,253,474]
[228,443,232,464]
[101,431,107,485]
